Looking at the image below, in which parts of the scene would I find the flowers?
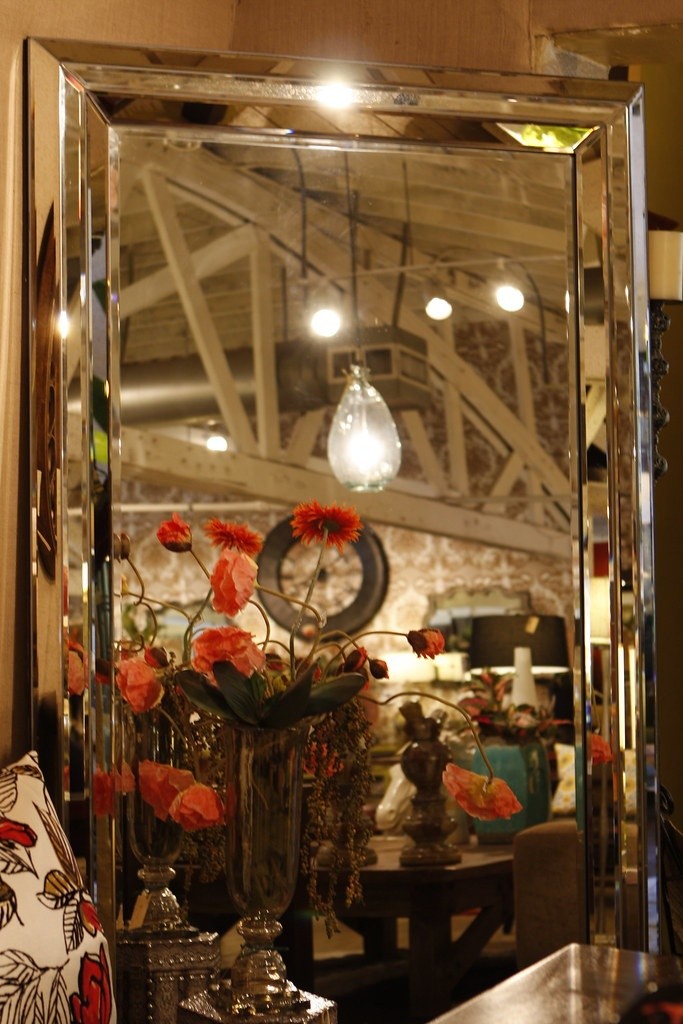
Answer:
[590,731,614,764]
[64,563,116,827]
[117,499,522,833]
[457,665,571,741]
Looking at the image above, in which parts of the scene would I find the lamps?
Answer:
[421,272,453,322]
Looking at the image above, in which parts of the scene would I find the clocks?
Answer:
[251,510,388,644]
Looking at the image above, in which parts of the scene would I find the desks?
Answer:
[316,840,515,1024]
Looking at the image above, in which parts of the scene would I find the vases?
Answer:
[473,736,553,845]
[122,703,201,940]
[207,722,310,1018]
[513,647,538,710]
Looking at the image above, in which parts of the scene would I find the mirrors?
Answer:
[23,34,663,1024]
[421,583,533,651]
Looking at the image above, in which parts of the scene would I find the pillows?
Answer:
[0,750,117,1024]
